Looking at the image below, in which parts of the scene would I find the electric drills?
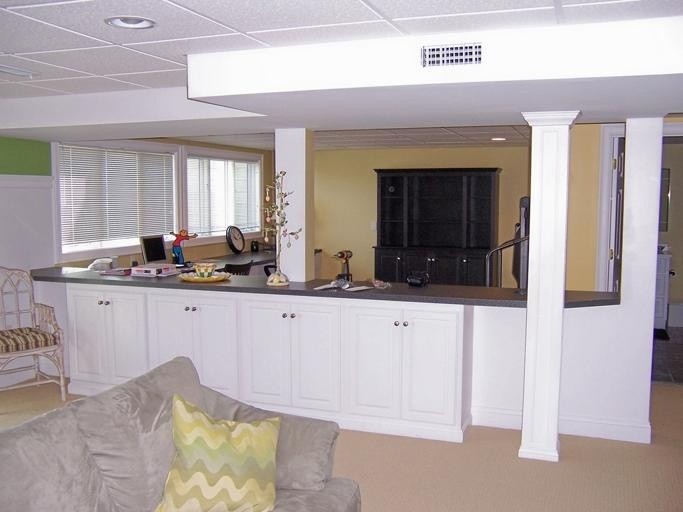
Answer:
[332,250,353,282]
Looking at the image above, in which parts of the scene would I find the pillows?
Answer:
[154,396,281,512]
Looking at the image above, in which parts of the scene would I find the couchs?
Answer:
[0,356,361,512]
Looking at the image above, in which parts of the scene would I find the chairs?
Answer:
[0,267,66,402]
[224,260,253,276]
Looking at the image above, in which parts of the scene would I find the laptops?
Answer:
[140,235,185,268]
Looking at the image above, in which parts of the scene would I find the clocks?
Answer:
[226,226,245,253]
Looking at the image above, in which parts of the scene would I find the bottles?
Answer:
[250,240,258,251]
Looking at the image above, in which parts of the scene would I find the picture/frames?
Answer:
[659,168,670,232]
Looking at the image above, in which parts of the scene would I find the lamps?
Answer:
[264,170,301,286]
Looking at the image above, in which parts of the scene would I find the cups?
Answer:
[193,263,215,278]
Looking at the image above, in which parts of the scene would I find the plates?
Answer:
[177,272,232,283]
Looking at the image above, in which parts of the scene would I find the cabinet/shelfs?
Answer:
[372,167,502,287]
[67,283,147,398]
[147,287,238,399]
[341,297,473,444]
[654,254,672,330]
[239,291,341,426]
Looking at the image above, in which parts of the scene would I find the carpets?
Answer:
[651,327,683,385]
